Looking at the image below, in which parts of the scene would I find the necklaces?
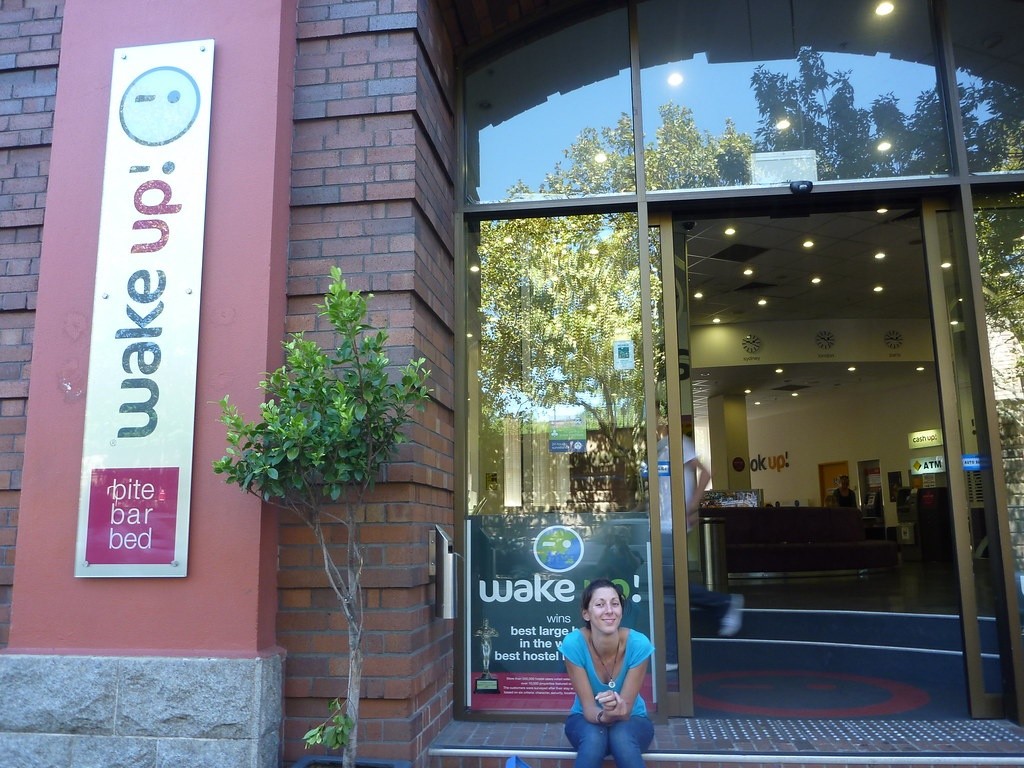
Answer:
[592,640,619,688]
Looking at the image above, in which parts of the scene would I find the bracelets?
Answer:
[595,710,604,735]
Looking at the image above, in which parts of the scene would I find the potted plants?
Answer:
[207,266,422,768]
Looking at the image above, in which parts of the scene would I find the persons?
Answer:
[557,579,655,768]
[599,526,644,629]
[832,476,858,509]
[642,399,744,671]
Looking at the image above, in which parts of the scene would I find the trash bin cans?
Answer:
[699,517,728,591]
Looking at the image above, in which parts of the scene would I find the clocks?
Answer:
[741,334,761,353]
[884,328,904,349]
[815,329,835,350]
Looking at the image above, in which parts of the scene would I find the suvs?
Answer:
[578,516,682,576]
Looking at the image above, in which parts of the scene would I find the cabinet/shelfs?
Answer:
[917,485,954,566]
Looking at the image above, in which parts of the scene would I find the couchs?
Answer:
[701,506,900,576]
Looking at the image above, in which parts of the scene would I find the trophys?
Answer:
[473,618,500,693]
